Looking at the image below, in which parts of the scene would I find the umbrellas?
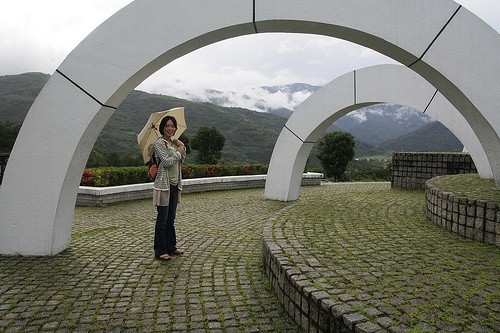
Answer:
[136,107,188,164]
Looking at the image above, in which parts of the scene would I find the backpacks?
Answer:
[148,153,161,181]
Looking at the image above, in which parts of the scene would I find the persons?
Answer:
[151,116,187,260]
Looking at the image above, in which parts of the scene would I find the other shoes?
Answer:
[160,253,171,259]
[173,248,184,254]
